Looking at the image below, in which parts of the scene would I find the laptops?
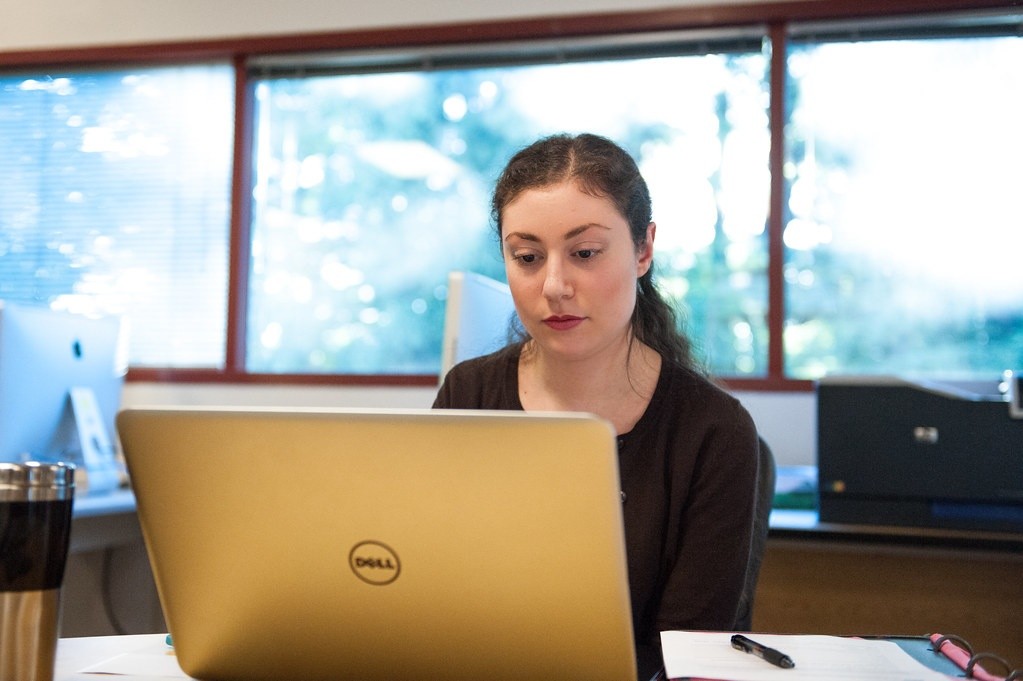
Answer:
[115,405,639,681]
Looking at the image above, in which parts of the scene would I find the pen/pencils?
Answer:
[730,634,795,670]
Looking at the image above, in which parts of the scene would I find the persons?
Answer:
[432,131,761,681]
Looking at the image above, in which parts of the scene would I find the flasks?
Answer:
[0,460,76,681]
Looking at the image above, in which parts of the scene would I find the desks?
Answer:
[67,488,142,553]
[53,633,200,681]
[760,508,1023,562]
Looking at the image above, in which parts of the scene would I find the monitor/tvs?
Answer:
[0,303,119,497]
[815,374,1023,535]
[443,271,529,391]
